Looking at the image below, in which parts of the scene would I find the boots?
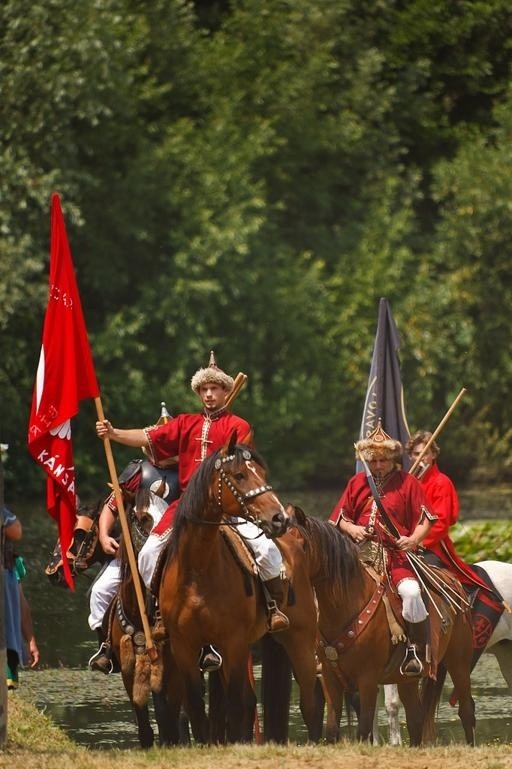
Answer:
[261,575,288,630]
[90,627,111,674]
[401,612,431,678]
[201,653,220,669]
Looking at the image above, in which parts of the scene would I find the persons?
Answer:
[84,397,223,672]
[325,418,441,677]
[2,500,43,693]
[401,427,493,593]
[94,349,292,642]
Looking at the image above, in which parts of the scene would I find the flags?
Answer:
[355,296,417,479]
[27,190,104,593]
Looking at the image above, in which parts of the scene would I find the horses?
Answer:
[275,504,475,748]
[153,425,320,739]
[373,559,512,749]
[102,463,171,750]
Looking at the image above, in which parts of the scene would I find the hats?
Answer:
[151,401,174,427]
[190,350,234,397]
[355,417,404,462]
